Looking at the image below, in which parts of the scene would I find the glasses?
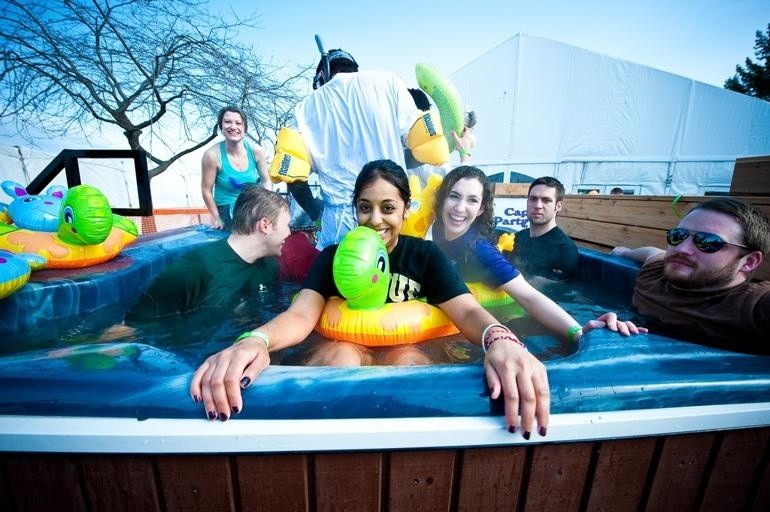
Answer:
[313,71,323,90]
[667,228,752,254]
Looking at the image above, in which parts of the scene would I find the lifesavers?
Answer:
[1,177,139,294]
[310,224,462,348]
[402,173,521,311]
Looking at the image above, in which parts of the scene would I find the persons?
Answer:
[269,49,449,254]
[99,187,291,342]
[190,161,550,441]
[611,188,624,195]
[503,176,579,289]
[547,197,770,357]
[431,126,649,345]
[201,106,272,230]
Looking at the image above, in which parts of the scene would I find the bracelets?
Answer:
[459,148,471,159]
[482,324,528,354]
[234,332,269,347]
[566,325,582,347]
